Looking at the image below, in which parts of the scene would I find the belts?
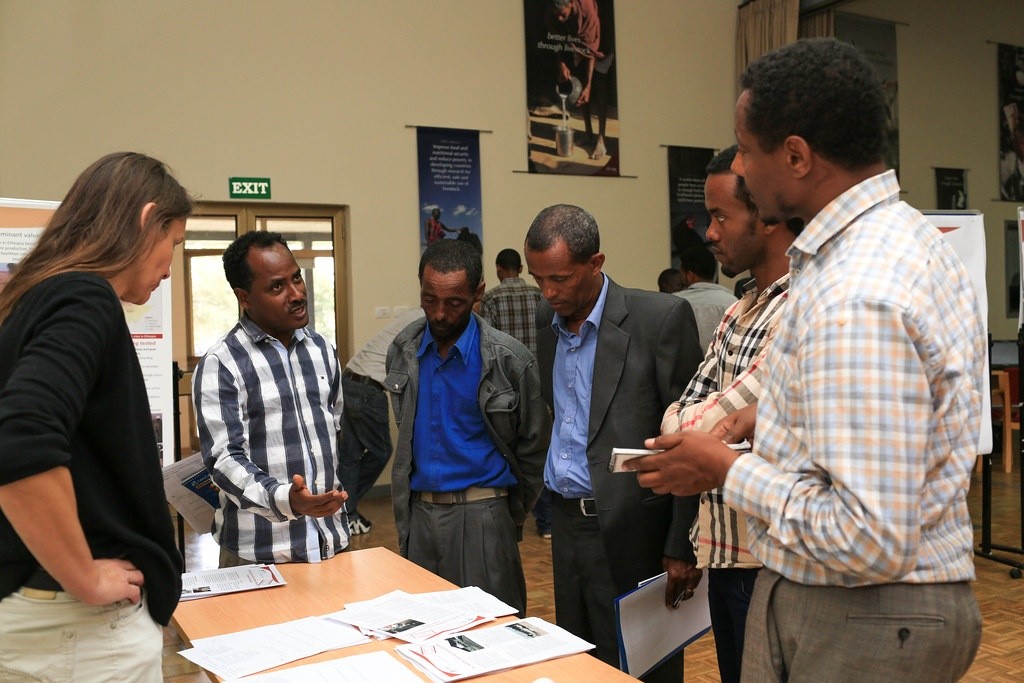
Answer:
[345,370,385,390]
[544,489,601,519]
[408,486,510,504]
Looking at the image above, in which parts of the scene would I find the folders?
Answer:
[614,563,713,680]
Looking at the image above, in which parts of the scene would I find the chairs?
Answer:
[976,370,1021,476]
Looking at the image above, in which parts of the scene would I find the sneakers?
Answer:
[348,516,371,536]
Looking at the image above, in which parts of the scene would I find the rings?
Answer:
[686,588,696,593]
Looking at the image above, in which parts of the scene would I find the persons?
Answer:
[657,246,753,358]
[425,208,464,254]
[382,239,553,620]
[525,203,705,683]
[542,0,614,160]
[673,216,716,278]
[337,308,425,536]
[621,37,985,683]
[191,231,350,569]
[0,152,193,683]
[660,144,803,683]
[480,249,553,538]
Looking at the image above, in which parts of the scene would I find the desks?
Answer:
[172,544,646,683]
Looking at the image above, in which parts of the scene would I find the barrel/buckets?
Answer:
[555,126,575,157]
[555,75,583,105]
